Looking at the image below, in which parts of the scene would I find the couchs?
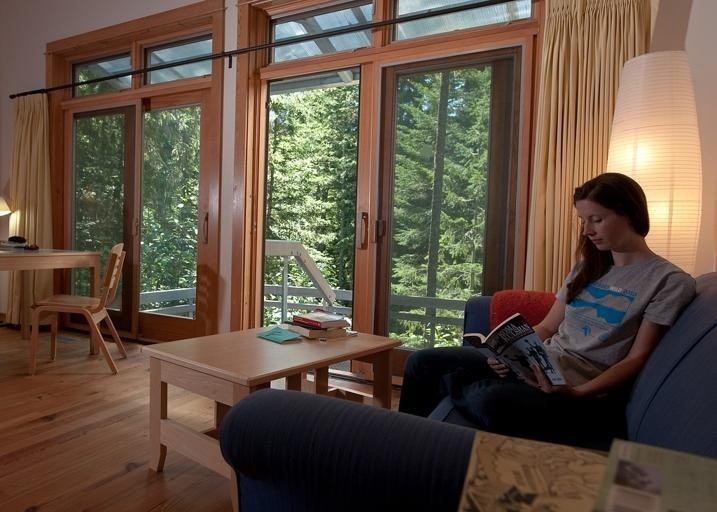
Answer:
[219,272,717,512]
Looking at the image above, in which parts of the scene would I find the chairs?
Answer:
[29,243,128,376]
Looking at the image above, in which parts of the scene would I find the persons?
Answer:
[397,170,697,453]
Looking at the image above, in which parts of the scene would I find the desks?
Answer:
[0,248,102,356]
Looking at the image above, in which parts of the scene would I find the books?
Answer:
[593,438,716,511]
[462,311,565,392]
[293,310,345,328]
[286,323,346,338]
[257,326,303,346]
[282,320,321,330]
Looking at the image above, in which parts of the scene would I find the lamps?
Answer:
[606,50,703,280]
[0,196,11,217]
